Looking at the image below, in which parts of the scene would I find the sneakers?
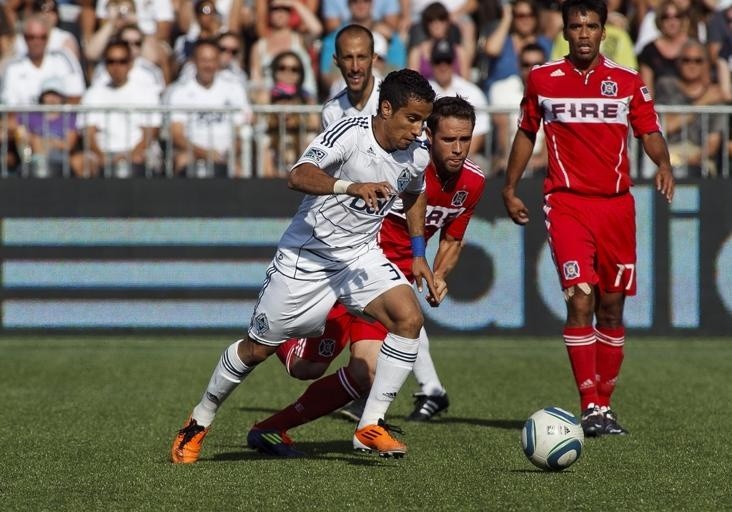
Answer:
[405,393,449,422]
[170,414,210,464]
[579,406,629,439]
[247,426,310,458]
[351,418,409,456]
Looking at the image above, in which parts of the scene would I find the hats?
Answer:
[373,32,388,57]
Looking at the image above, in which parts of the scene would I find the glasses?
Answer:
[21,0,302,73]
[663,12,704,65]
[426,13,545,68]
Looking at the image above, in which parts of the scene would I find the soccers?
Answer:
[521,407,584,471]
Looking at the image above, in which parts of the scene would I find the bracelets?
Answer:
[330,177,353,197]
[408,233,425,259]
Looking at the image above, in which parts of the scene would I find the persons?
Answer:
[0,2,732,183]
[246,96,486,458]
[320,22,448,419]
[502,0,677,436]
[173,68,443,463]
